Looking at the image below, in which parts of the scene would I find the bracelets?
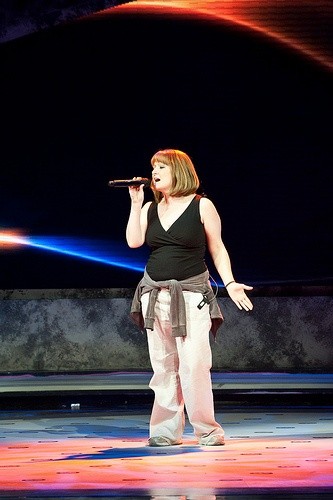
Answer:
[226,281,235,287]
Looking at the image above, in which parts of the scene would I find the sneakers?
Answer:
[148,435,225,446]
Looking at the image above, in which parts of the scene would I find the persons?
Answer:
[126,149,253,446]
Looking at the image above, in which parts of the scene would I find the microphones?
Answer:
[108,178,150,188]
